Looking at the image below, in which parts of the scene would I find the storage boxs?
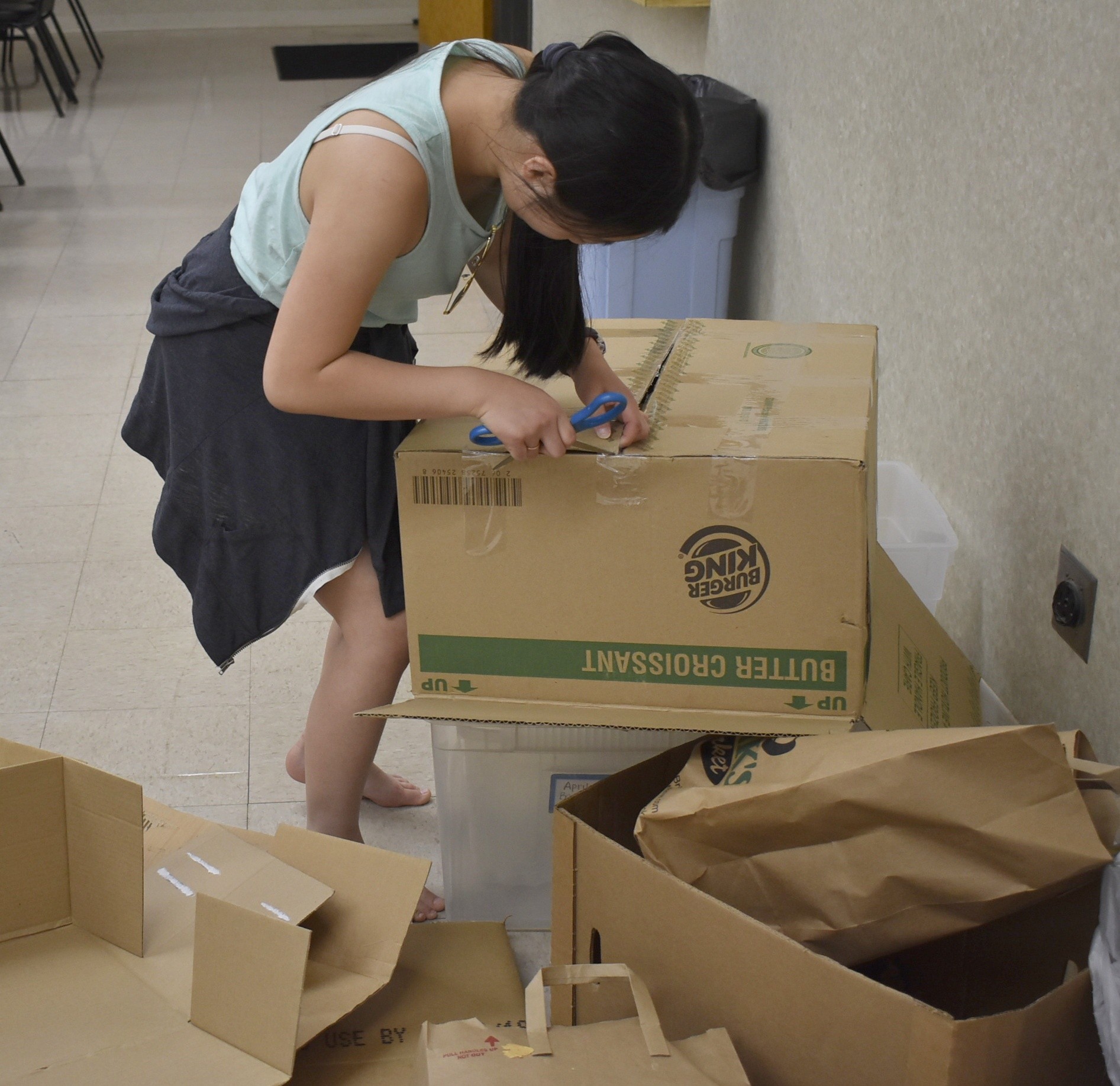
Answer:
[550,735,1095,1086]
[351,317,879,729]
[424,719,702,981]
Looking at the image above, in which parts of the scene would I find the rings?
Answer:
[527,444,540,451]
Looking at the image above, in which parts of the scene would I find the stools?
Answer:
[0,0,103,117]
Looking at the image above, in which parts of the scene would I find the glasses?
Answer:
[444,225,496,314]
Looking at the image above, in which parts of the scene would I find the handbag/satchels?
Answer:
[423,963,718,1086]
[633,712,1120,967]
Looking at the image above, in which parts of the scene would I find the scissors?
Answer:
[467,393,627,471]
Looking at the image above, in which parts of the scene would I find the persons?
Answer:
[120,30,698,924]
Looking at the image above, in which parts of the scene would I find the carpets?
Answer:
[270,41,419,80]
